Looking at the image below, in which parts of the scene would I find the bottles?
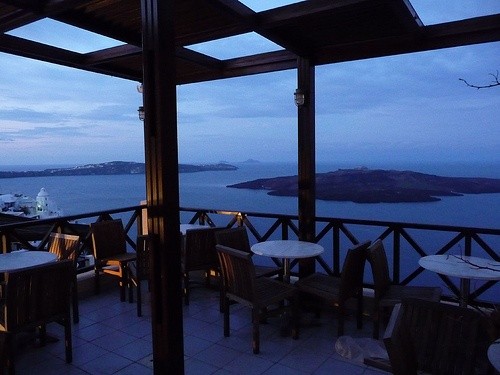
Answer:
[36,188,50,214]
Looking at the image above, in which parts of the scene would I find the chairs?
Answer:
[22,232,80,346]
[366,239,439,340]
[181,228,218,306]
[89,218,137,303]
[215,244,299,354]
[383,302,500,375]
[127,235,155,319]
[291,240,372,339]
[214,227,284,324]
[0,260,75,375]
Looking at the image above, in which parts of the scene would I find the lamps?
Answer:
[138,107,146,122]
[294,89,305,109]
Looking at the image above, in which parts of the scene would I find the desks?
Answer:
[418,254,500,308]
[180,224,212,236]
[1,250,58,347]
[250,239,325,321]
